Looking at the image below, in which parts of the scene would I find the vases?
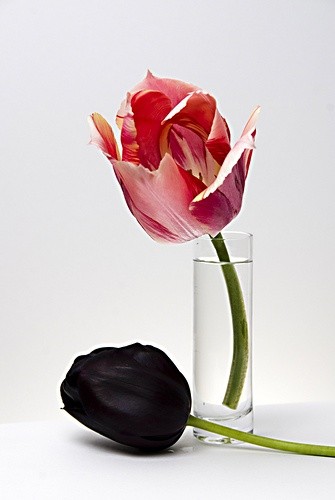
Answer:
[181,233,258,446]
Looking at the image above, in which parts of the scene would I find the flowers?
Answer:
[87,65,266,411]
[59,341,335,464]
